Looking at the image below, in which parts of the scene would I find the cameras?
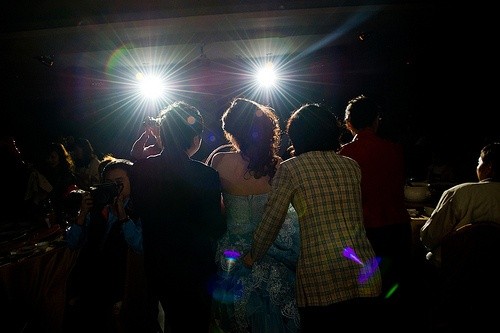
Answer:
[91,182,120,208]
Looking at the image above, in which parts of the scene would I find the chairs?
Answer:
[424,221,500,333]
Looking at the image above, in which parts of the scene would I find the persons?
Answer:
[418,143,500,268]
[243,103,389,333]
[0,132,163,333]
[126,108,228,333]
[335,94,413,333]
[209,104,301,333]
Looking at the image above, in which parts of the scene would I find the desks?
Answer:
[0,236,73,333]
